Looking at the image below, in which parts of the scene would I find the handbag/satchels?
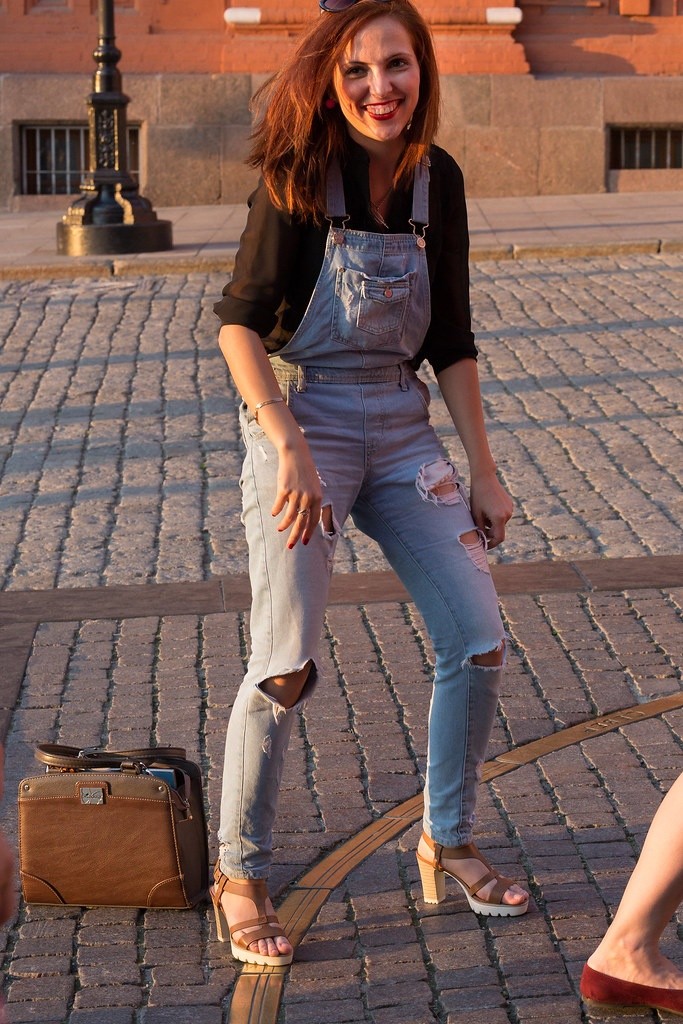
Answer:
[17,744,210,910]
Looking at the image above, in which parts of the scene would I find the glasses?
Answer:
[319,0,361,13]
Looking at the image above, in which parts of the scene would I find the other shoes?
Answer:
[580,961,683,1016]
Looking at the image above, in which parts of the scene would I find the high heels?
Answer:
[209,858,293,966]
[415,831,529,917]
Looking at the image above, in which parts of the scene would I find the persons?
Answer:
[580,769,683,1015]
[206,0,529,964]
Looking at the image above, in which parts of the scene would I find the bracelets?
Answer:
[253,396,285,427]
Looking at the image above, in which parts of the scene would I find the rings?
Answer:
[297,509,310,517]
